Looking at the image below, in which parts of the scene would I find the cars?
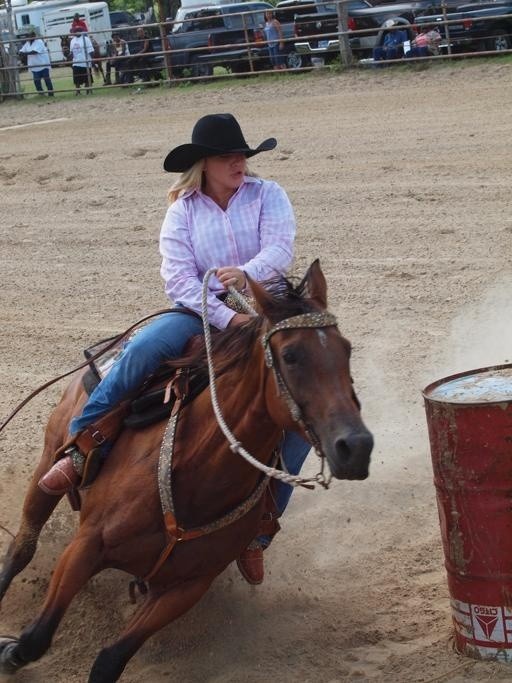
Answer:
[275,0,375,68]
[108,10,146,42]
[413,0,512,57]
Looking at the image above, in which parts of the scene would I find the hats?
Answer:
[111,32,121,38]
[163,113,278,173]
[73,25,83,33]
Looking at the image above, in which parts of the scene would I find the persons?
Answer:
[373,17,410,69]
[406,19,442,65]
[126,26,163,86]
[68,25,98,96]
[262,10,289,75]
[38,109,315,588]
[101,30,131,85]
[15,31,54,96]
[69,12,88,37]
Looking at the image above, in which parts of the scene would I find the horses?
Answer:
[0,258,373,683]
[60,35,101,77]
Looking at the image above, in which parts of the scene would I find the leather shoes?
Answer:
[236,539,265,585]
[38,451,83,496]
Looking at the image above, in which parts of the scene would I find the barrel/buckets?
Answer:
[419,364,512,664]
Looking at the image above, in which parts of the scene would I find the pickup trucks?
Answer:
[105,1,310,87]
[293,0,434,60]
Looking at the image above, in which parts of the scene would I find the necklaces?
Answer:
[216,190,235,206]
